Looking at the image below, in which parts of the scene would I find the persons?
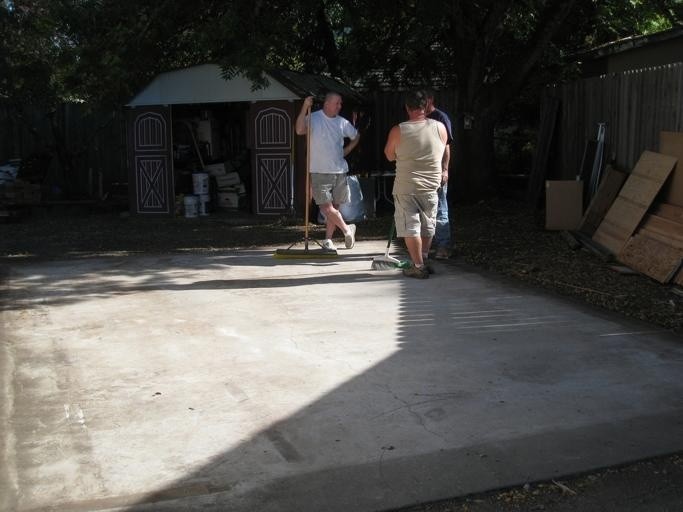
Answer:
[420,89,454,262]
[293,89,360,251]
[384,88,447,281]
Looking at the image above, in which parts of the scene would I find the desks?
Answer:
[368,171,397,206]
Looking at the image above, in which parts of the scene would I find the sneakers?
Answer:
[323,239,337,251]
[345,224,356,248]
[435,247,449,259]
[403,258,434,279]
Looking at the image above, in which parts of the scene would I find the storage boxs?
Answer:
[207,164,247,210]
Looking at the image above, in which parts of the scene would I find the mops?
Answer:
[274,97,338,259]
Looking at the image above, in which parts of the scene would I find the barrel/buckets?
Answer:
[199,195,211,216]
[184,195,197,217]
[192,173,209,194]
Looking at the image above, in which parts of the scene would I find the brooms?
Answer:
[372,218,401,269]
